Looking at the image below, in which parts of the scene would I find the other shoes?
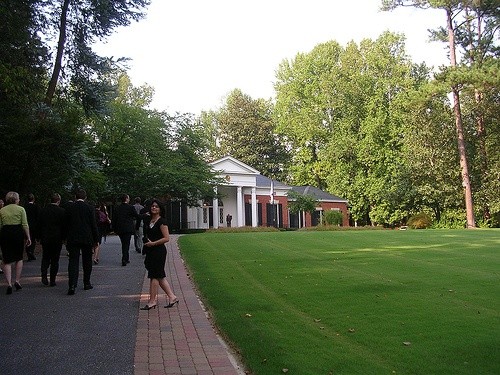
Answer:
[0,269,4,274]
[68,288,75,295]
[122,260,126,266]
[125,260,130,263]
[14,281,23,289]
[93,257,100,264]
[28,253,36,262]
[42,277,48,285]
[50,283,56,286]
[6,286,12,294]
[84,286,93,290]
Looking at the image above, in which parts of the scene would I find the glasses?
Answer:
[151,205,159,208]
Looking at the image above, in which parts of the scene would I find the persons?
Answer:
[138,198,180,311]
[0,188,151,296]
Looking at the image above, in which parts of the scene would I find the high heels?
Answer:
[164,299,179,308]
[141,304,156,310]
